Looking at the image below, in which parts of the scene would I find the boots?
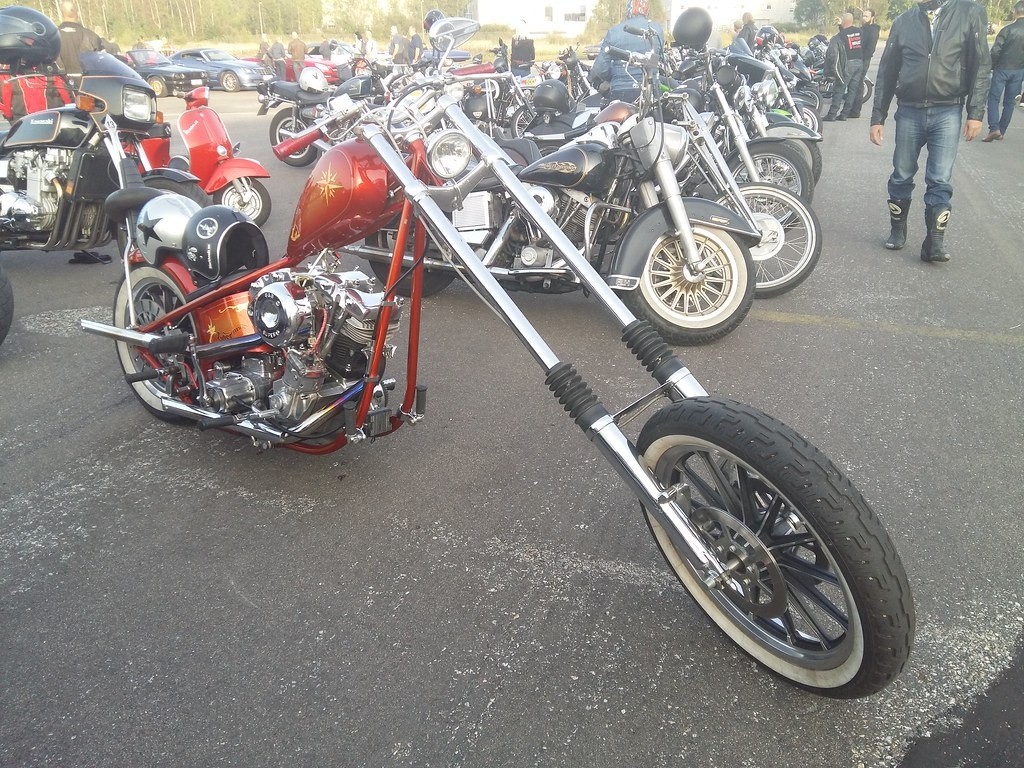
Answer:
[921,202,951,262]
[821,105,837,121]
[886,198,912,249]
[837,107,851,121]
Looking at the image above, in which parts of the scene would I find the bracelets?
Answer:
[414,58,417,60]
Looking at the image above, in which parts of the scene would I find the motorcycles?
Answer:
[258,11,873,344]
[80,18,915,699]
[0,5,211,257]
[124,86,272,228]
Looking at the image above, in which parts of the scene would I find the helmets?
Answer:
[181,205,269,288]
[1,7,61,69]
[755,26,779,47]
[425,10,442,30]
[136,194,203,266]
[809,38,819,49]
[673,8,713,50]
[534,80,573,116]
[299,66,328,95]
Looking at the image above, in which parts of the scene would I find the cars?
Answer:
[126,47,273,98]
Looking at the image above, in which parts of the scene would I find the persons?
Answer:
[52,0,130,90]
[407,24,424,66]
[868,1,992,262]
[288,33,308,61]
[269,37,286,68]
[982,0,1024,143]
[511,20,535,77]
[353,32,365,56]
[388,26,404,64]
[737,12,759,56]
[94,25,121,55]
[592,2,664,105]
[318,34,332,61]
[364,31,378,60]
[846,9,880,118]
[132,34,172,57]
[723,20,743,54]
[821,13,865,121]
[257,34,270,57]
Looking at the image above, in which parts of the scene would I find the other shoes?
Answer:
[848,111,860,119]
[982,131,1004,140]
[1015,95,1024,107]
